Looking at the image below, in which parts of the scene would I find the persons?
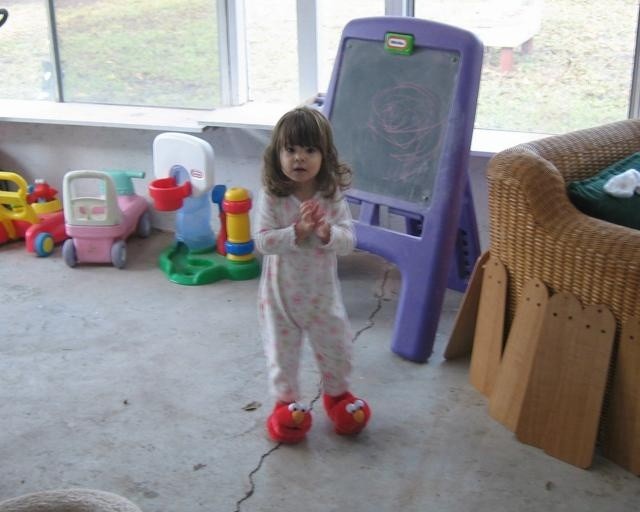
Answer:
[255,107,371,442]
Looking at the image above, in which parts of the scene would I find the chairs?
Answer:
[487,117,640,441]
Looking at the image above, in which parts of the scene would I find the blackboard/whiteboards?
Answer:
[330,39,461,207]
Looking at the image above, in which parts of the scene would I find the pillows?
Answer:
[567,150,640,230]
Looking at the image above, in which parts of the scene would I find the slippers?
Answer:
[324,389,371,436]
[268,399,312,444]
